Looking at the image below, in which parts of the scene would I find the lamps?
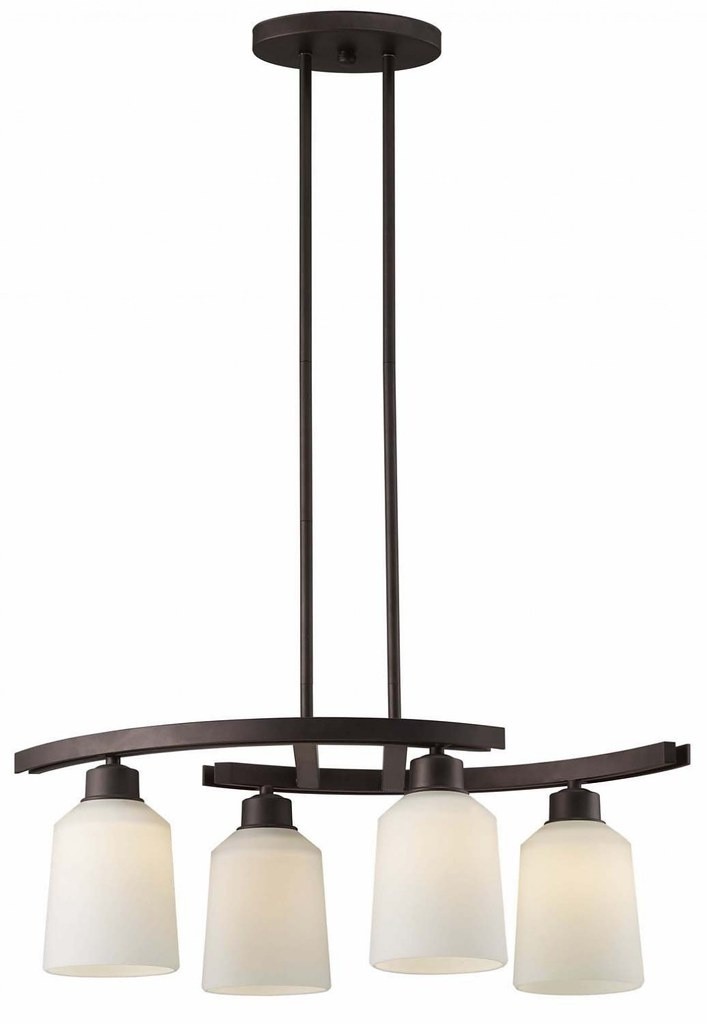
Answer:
[10,7,691,994]
